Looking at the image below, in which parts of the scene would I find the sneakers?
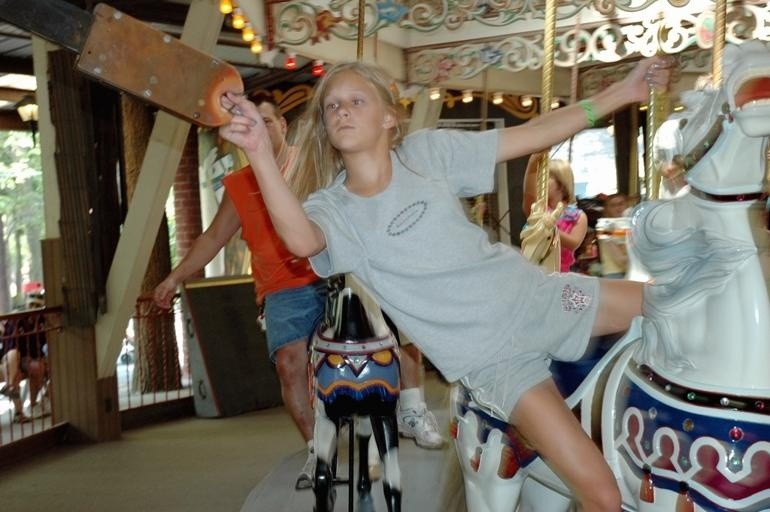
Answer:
[397,404,444,449]
[295,449,317,489]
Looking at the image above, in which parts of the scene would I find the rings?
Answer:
[234,108,242,117]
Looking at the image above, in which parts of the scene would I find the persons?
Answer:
[522,150,588,269]
[6,287,52,417]
[217,56,671,512]
[594,192,636,280]
[0,322,29,425]
[151,91,445,489]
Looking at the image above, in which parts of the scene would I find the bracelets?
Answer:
[578,99,597,128]
